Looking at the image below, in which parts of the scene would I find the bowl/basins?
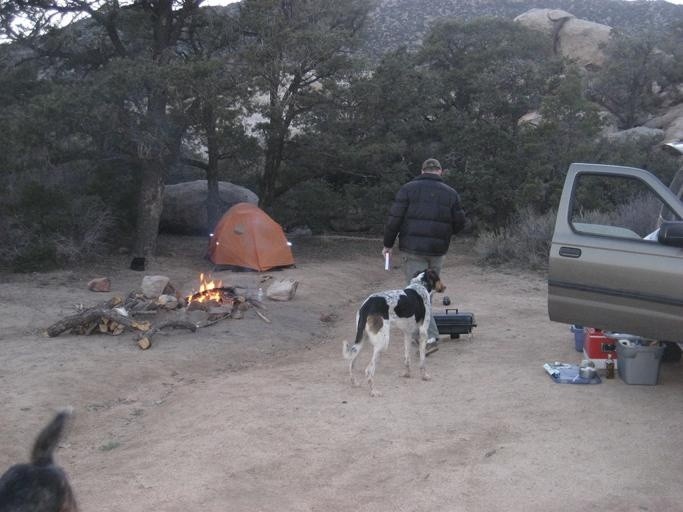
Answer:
[580,367,596,379]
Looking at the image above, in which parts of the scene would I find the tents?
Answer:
[204,202,296,272]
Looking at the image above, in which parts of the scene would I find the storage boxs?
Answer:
[584,330,617,358]
[571,325,585,352]
[614,338,667,385]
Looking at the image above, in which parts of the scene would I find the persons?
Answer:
[383,158,464,356]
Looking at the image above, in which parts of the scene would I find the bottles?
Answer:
[258,287,263,301]
[606,355,614,379]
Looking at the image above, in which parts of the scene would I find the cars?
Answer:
[548,140,682,355]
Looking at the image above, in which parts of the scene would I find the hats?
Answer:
[422,159,441,170]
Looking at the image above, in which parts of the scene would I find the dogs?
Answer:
[342,267,447,397]
[0,405,79,512]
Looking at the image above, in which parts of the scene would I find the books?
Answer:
[385,253,389,270]
